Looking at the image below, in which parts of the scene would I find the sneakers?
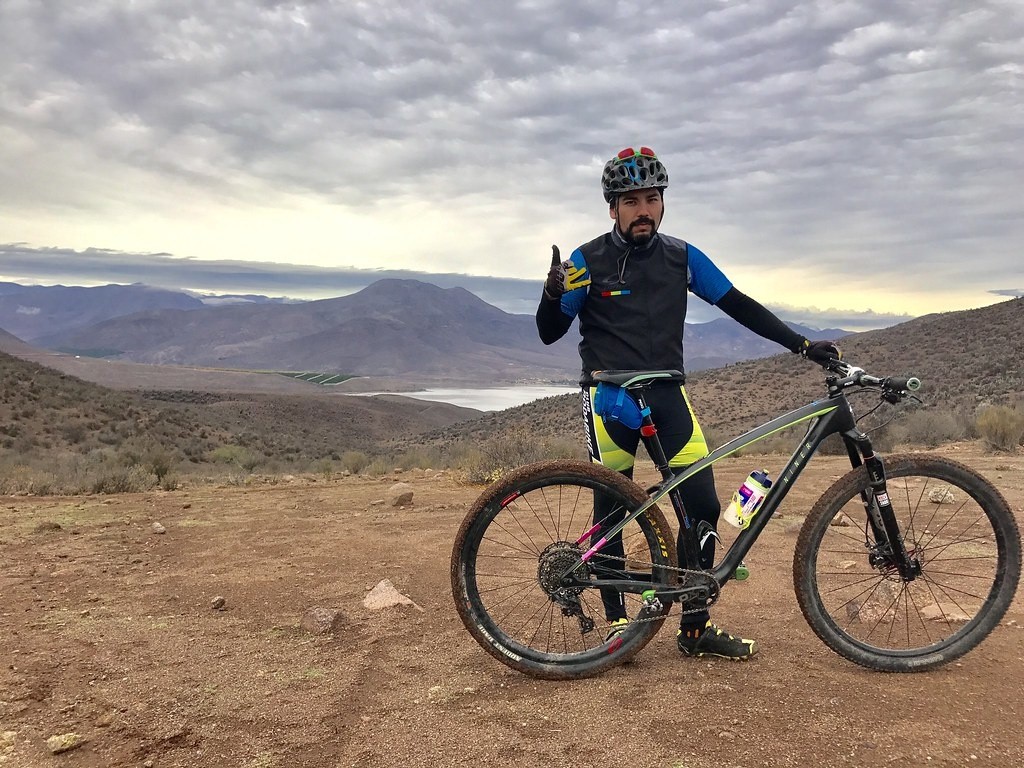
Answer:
[678,622,758,661]
[606,618,628,641]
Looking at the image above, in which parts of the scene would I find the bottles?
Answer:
[723,469,773,529]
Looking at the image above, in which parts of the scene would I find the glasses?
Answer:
[615,147,657,162]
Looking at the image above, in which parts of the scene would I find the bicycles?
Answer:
[448,355,1022,678]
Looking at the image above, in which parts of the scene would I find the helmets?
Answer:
[601,151,669,203]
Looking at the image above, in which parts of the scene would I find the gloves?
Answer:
[801,338,842,367]
[546,245,592,298]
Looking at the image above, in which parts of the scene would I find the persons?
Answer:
[535,147,843,660]
